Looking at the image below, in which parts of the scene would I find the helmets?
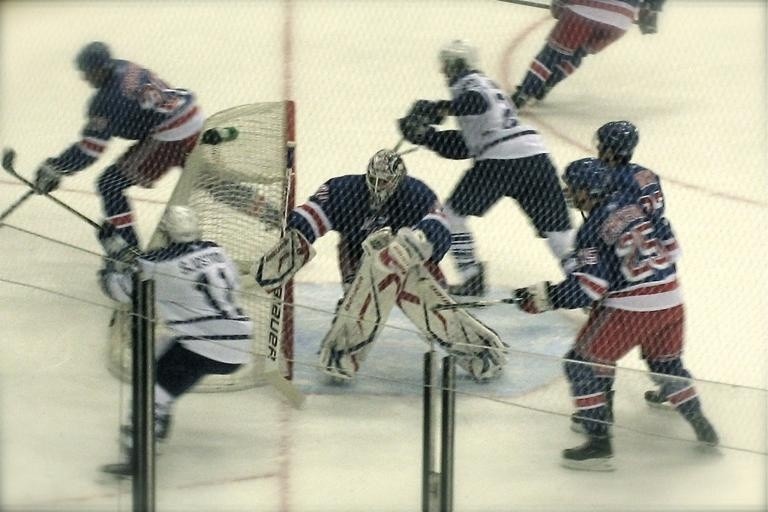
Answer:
[561,157,612,188]
[164,206,200,242]
[438,42,481,87]
[366,148,405,204]
[597,121,637,153]
[75,41,111,86]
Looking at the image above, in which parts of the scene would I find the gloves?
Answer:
[34,158,59,195]
[515,282,554,314]
[398,100,449,143]
[374,227,432,272]
[98,220,129,258]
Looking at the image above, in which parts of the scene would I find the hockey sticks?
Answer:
[2,149,102,231]
[429,299,523,312]
[392,135,418,156]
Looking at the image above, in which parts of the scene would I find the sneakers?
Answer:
[121,413,167,439]
[100,463,135,475]
[563,386,716,460]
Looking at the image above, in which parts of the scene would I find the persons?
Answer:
[32,41,286,245]
[399,39,596,316]
[569,120,682,435]
[515,158,719,472]
[280,148,453,330]
[99,205,255,477]
[511,0,665,115]
